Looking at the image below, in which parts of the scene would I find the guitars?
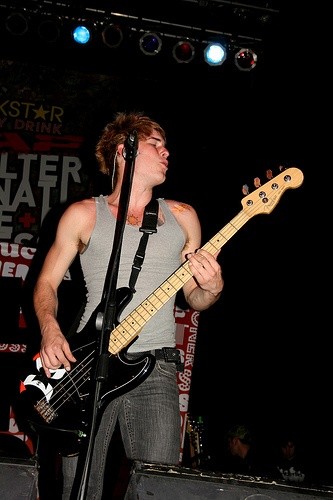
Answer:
[10,161,305,457]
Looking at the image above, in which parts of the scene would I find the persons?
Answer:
[33,114,224,500]
[225,425,274,478]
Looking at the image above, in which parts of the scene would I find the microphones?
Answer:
[122,128,137,159]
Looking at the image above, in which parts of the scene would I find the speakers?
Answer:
[123,461,333,500]
[0,456,39,500]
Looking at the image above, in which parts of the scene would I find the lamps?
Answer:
[100,25,124,48]
[202,42,227,66]
[171,40,196,64]
[70,21,97,47]
[231,46,258,71]
[4,10,36,36]
[138,32,164,57]
